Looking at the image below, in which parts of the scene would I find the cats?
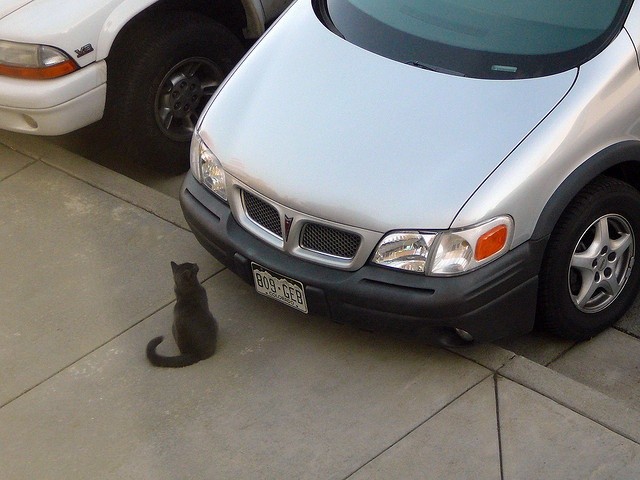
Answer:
[142,259,220,369]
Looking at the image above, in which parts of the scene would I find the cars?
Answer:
[0,0,295,177]
[178,0,640,349]
